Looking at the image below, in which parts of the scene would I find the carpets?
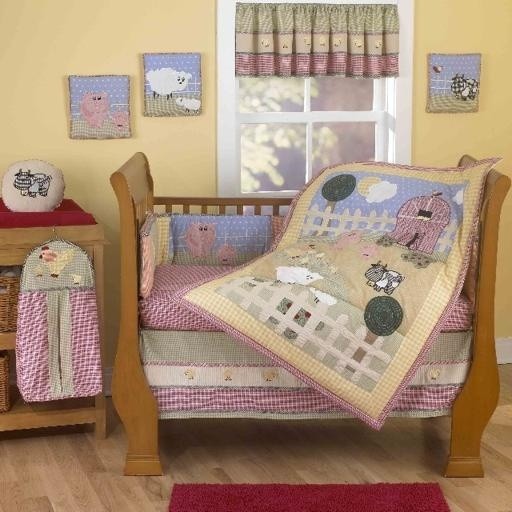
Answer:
[167,483,452,512]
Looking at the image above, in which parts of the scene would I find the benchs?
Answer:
[108,152,512,478]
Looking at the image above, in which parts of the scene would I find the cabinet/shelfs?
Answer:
[0,199,112,440]
